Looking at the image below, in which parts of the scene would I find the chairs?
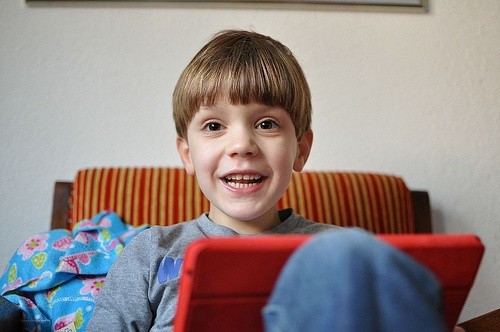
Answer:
[50,166,431,234]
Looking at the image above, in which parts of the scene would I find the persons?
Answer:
[87,29,465,331]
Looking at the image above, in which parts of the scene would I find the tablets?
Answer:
[173,234,485,332]
